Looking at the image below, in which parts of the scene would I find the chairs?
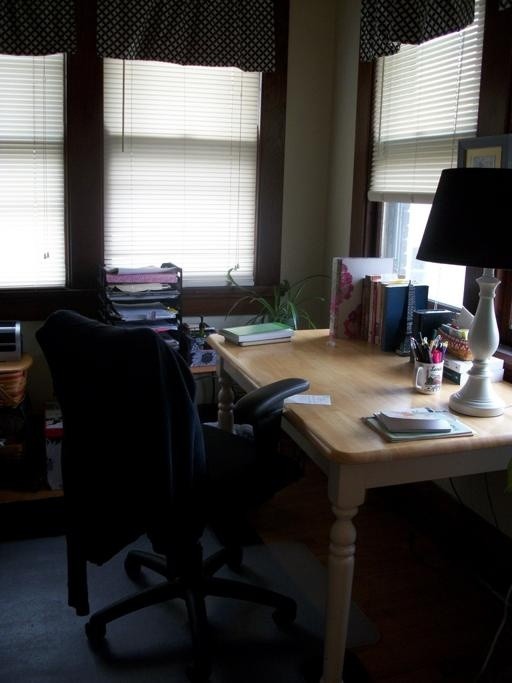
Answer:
[34,309,311,683]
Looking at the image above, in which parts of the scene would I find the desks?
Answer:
[202,329,512,683]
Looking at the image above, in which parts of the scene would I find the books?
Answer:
[225,335,292,347]
[220,320,292,342]
[326,254,505,385]
[359,408,474,442]
[373,405,451,434]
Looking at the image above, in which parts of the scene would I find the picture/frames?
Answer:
[457,133,511,169]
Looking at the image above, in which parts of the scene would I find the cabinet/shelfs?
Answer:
[95,263,190,368]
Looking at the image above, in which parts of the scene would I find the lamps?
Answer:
[416,166,512,419]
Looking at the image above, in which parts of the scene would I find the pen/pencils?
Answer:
[409,328,449,363]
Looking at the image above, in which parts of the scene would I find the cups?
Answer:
[413,356,445,395]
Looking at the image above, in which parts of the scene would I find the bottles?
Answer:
[199,316,206,336]
